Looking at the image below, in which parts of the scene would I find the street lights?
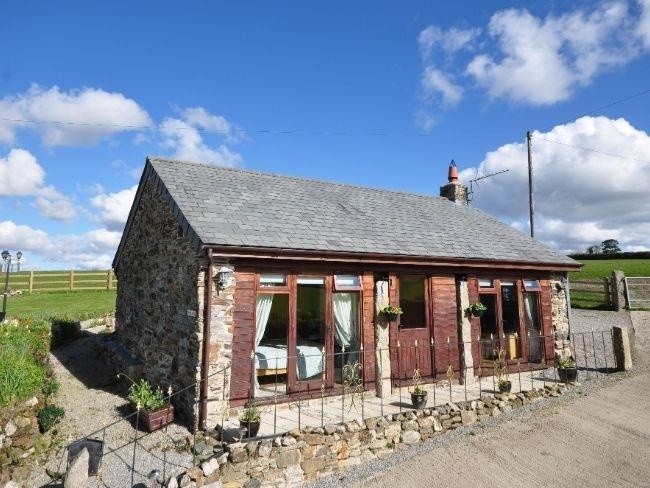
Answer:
[0,249,22,320]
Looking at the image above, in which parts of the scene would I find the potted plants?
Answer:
[467,302,488,318]
[556,357,577,383]
[379,304,404,322]
[499,380,512,394]
[408,386,429,410]
[237,398,270,439]
[113,372,176,433]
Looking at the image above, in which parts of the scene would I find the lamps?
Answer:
[216,266,231,290]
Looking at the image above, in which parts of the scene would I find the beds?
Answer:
[254,338,326,387]
